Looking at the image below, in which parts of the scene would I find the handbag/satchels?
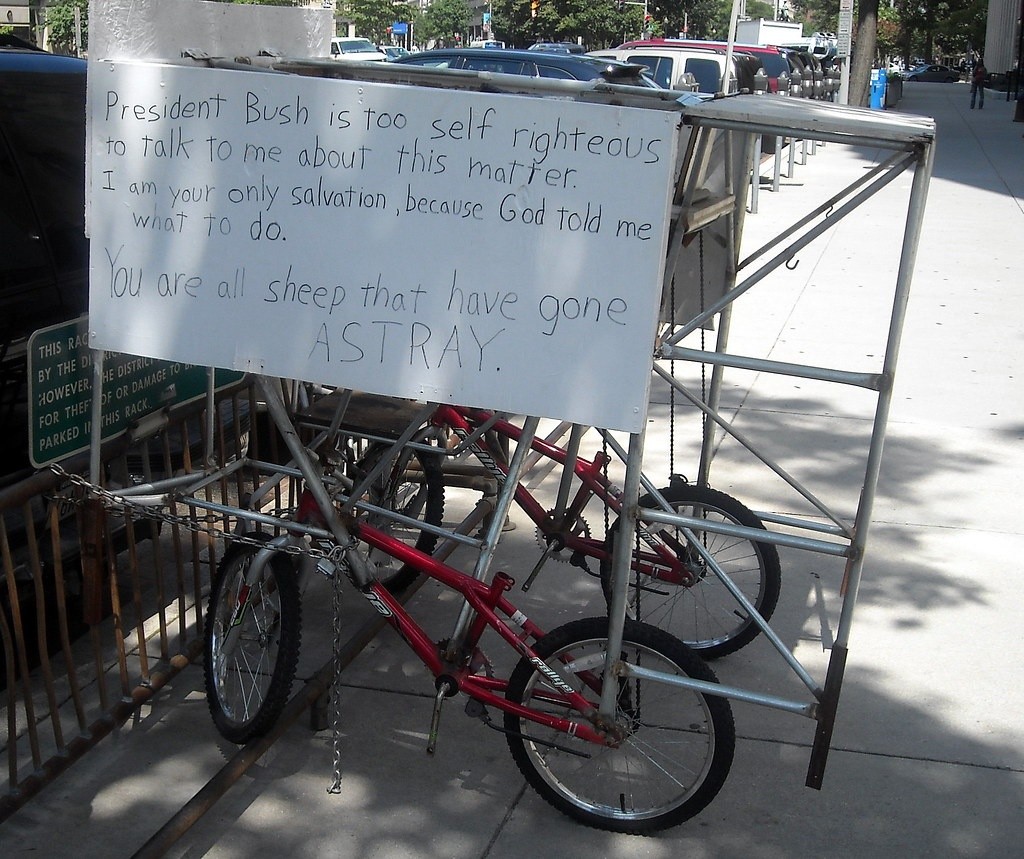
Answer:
[970,83,975,93]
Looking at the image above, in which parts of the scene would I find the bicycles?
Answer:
[341,399,782,662]
[204,390,736,835]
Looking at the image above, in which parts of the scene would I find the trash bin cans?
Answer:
[869,70,887,111]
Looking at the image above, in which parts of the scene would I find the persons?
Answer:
[961,59,967,73]
[969,59,987,109]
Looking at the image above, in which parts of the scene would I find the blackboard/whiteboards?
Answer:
[89,58,682,435]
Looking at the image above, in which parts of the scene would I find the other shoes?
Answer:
[979,105,982,108]
[970,105,974,109]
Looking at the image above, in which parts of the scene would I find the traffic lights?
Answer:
[644,15,651,31]
[619,0,624,11]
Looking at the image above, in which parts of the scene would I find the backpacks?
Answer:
[975,66,986,79]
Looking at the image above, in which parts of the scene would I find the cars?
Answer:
[871,55,966,84]
[0,45,90,361]
[329,32,837,99]
